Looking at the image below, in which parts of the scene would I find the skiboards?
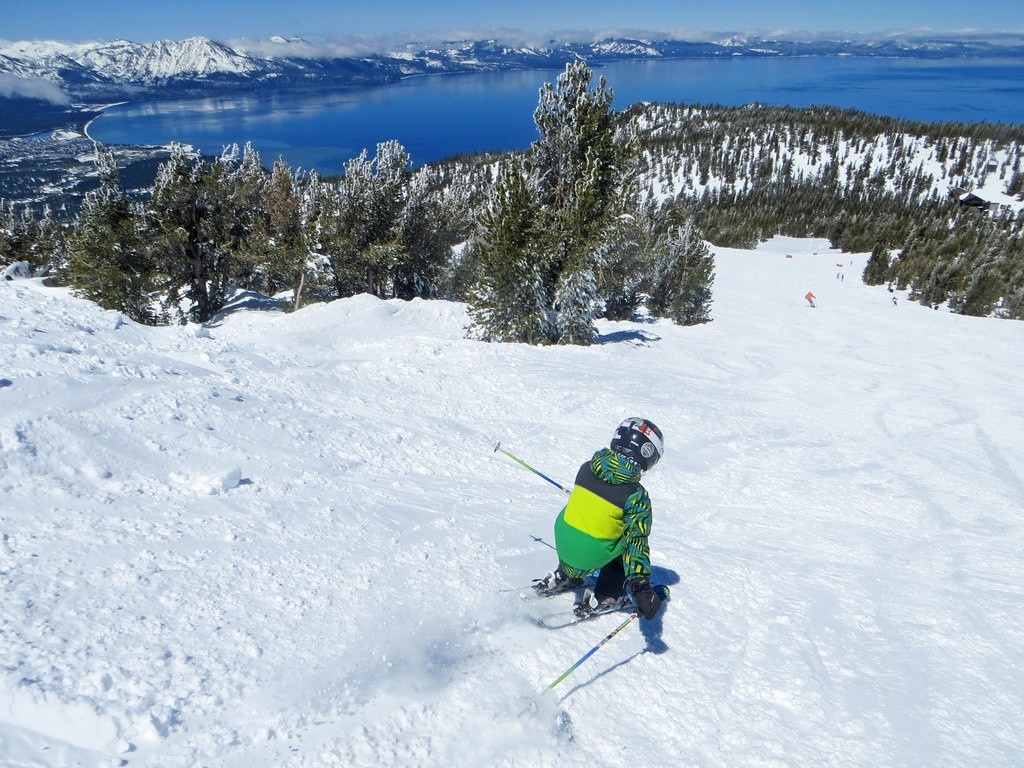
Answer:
[494,572,671,630]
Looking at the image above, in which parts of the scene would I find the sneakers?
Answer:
[535,569,584,595]
[589,592,632,618]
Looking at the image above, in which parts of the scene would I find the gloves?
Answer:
[631,577,661,620]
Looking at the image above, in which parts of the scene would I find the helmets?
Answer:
[610,417,665,472]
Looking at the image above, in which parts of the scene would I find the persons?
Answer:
[535,416,664,619]
